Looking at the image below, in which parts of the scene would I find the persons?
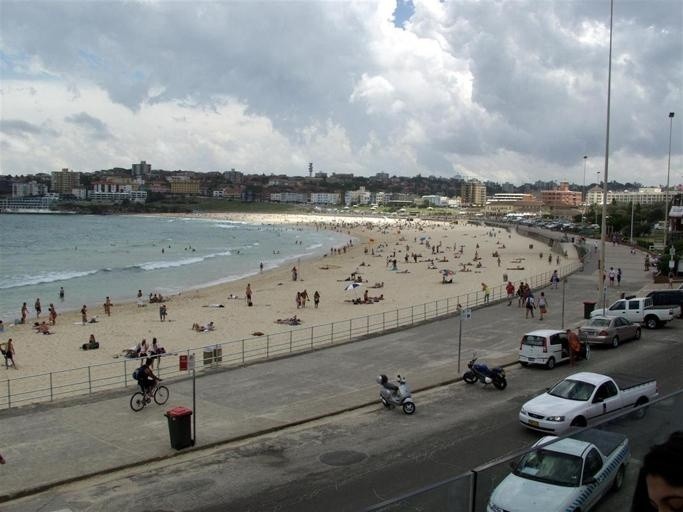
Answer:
[548,226,598,263]
[645,253,650,271]
[138,338,162,394]
[566,328,581,365]
[552,270,558,289]
[245,284,252,302]
[20,286,65,334]
[507,281,548,320]
[644,431,682,512]
[261,220,506,324]
[603,266,622,288]
[669,270,674,286]
[4,338,17,369]
[613,231,622,245]
[192,321,214,331]
[135,289,167,321]
[81,297,111,349]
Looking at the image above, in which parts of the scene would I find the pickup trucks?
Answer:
[518,371,659,435]
[589,295,681,330]
[485,425,631,511]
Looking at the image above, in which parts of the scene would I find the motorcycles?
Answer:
[462,352,506,390]
[376,374,415,414]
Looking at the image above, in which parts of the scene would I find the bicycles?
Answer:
[130,376,169,412]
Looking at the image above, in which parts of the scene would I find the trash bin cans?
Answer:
[164,405,194,450]
[583,301,597,319]
[203,345,222,364]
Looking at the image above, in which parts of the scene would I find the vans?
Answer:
[645,289,683,319]
[517,329,590,370]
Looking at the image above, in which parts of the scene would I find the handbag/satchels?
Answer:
[132,368,142,380]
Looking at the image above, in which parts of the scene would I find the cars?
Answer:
[575,315,641,348]
[458,210,601,233]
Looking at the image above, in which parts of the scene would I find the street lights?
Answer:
[622,188,634,243]
[662,111,675,247]
[594,171,600,225]
[580,155,587,207]
